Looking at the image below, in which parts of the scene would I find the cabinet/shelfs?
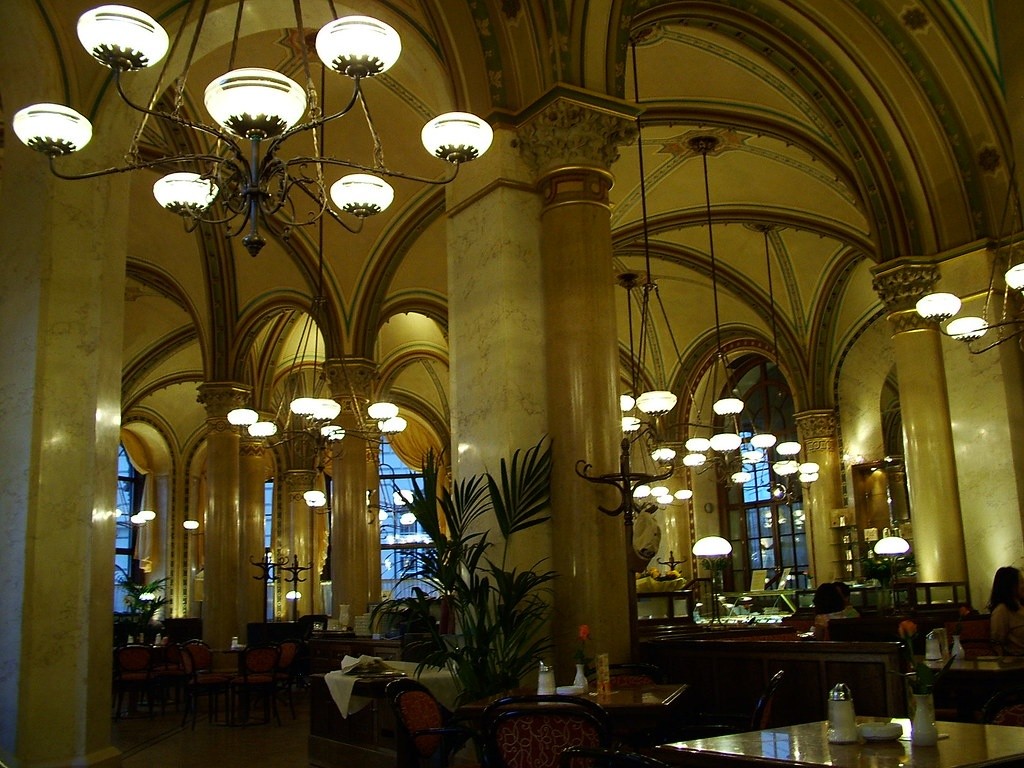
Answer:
[830,525,863,580]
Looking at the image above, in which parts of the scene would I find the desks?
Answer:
[210,646,281,727]
[657,717,1024,768]
[461,683,690,735]
[307,673,405,767]
[914,655,1024,672]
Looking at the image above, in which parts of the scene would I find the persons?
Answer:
[983,567,1024,723]
[815,582,858,641]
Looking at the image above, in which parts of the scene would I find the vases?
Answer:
[911,694,938,746]
[952,635,965,659]
[574,664,588,692]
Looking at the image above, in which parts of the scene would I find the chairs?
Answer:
[664,669,786,745]
[113,639,303,731]
[981,686,1024,727]
[584,664,662,684]
[385,679,670,768]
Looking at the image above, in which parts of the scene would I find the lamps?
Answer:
[183,520,204,536]
[228,65,407,472]
[693,535,733,629]
[619,37,820,504]
[916,163,1024,357]
[116,509,157,531]
[14,1,494,258]
[303,438,417,539]
[874,537,909,615]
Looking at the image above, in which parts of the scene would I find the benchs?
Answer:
[944,614,997,656]
[827,610,979,655]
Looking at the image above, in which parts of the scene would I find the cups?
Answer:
[327,619,338,631]
[338,604,350,630]
[313,622,323,632]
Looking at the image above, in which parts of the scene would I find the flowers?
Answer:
[635,567,681,582]
[957,606,970,635]
[575,625,593,664]
[887,619,957,694]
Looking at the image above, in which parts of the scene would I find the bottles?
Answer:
[828,683,858,744]
[231,635,239,651]
[155,633,168,646]
[925,630,942,661]
[594,642,610,694]
[537,660,556,705]
